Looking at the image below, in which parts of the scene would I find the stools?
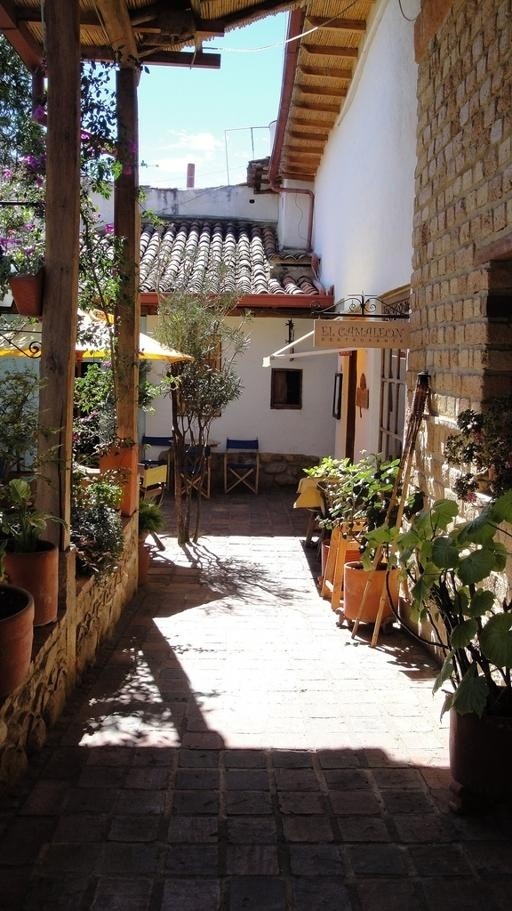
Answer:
[306,507,322,547]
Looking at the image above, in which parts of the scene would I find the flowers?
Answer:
[1,224,47,276]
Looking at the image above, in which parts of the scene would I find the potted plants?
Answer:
[1,477,58,627]
[138,496,164,543]
[0,539,35,696]
[344,492,423,624]
[390,492,512,820]
[310,454,405,585]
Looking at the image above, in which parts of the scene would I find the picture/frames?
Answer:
[332,372,344,419]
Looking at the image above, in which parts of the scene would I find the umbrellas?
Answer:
[0,306,195,465]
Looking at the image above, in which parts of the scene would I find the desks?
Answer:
[168,438,218,499]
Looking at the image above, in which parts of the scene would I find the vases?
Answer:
[7,267,43,317]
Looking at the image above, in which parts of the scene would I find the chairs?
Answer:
[223,432,260,495]
[139,435,176,467]
